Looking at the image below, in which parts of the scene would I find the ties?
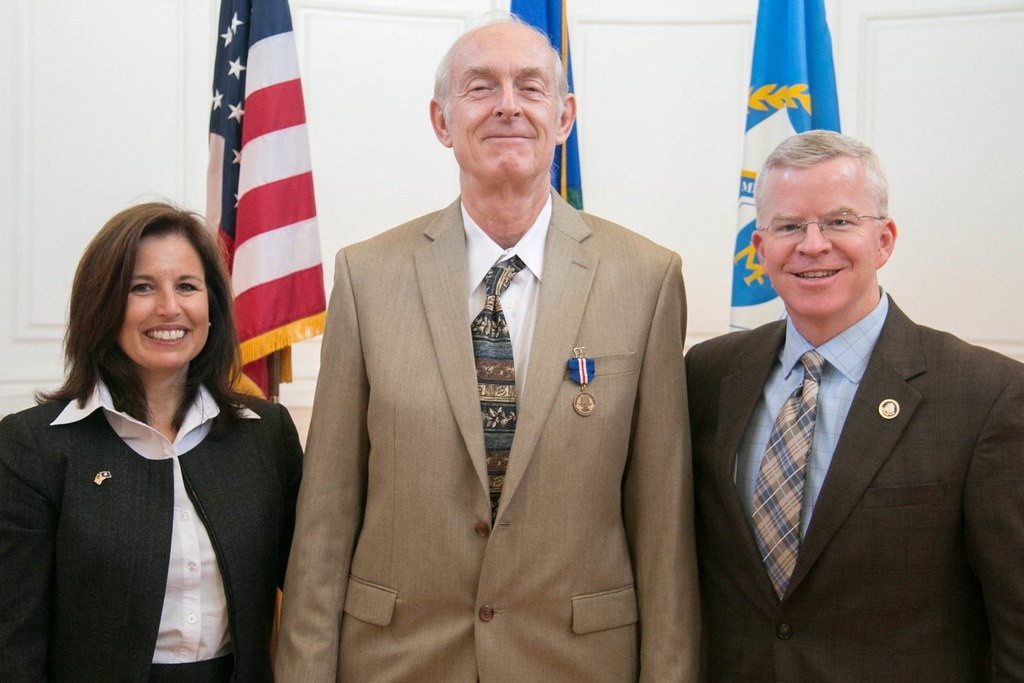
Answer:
[470,256,526,529]
[752,352,825,604]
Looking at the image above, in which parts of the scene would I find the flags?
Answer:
[203,0,327,402]
[510,0,583,210]
[730,0,841,330]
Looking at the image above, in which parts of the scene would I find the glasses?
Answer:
[759,210,884,241]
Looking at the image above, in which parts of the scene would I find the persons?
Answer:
[684,131,1024,683]
[275,23,701,683]
[0,202,303,683]
